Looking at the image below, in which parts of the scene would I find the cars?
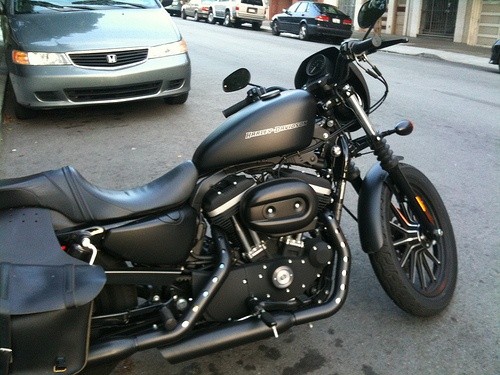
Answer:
[489,39,500,71]
[159,0,181,16]
[180,0,211,22]
[270,1,354,46]
[0,0,192,120]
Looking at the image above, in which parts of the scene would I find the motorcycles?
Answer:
[1,0,460,375]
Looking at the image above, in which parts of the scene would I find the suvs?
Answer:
[208,0,266,30]
